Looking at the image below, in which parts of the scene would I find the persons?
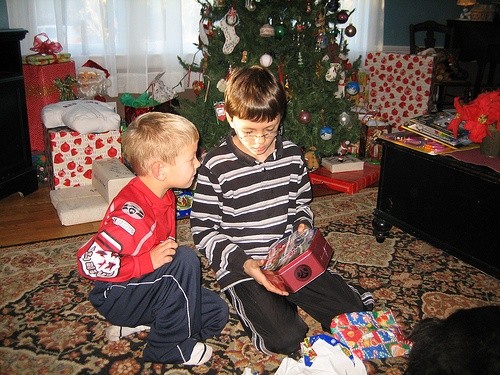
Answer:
[77,60,111,103]
[338,143,347,162]
[76,112,229,365]
[406,305,500,375]
[189,66,374,355]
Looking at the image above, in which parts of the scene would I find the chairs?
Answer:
[408,20,481,113]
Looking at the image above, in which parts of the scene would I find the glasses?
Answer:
[231,119,284,142]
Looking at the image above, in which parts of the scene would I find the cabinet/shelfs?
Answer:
[0,27,39,198]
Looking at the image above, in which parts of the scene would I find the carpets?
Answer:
[0,186,500,375]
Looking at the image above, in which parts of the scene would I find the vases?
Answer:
[480,130,500,155]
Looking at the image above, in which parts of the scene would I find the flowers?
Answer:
[447,90,500,143]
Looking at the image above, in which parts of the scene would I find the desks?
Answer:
[441,19,500,104]
[372,139,500,281]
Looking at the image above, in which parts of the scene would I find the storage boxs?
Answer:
[321,155,364,174]
[358,114,391,160]
[364,51,439,133]
[22,53,137,227]
[174,190,194,219]
[308,163,380,195]
[262,227,334,293]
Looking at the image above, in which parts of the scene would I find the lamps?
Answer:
[457,0,477,20]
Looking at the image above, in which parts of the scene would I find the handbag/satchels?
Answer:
[363,47,437,120]
[124,83,181,130]
